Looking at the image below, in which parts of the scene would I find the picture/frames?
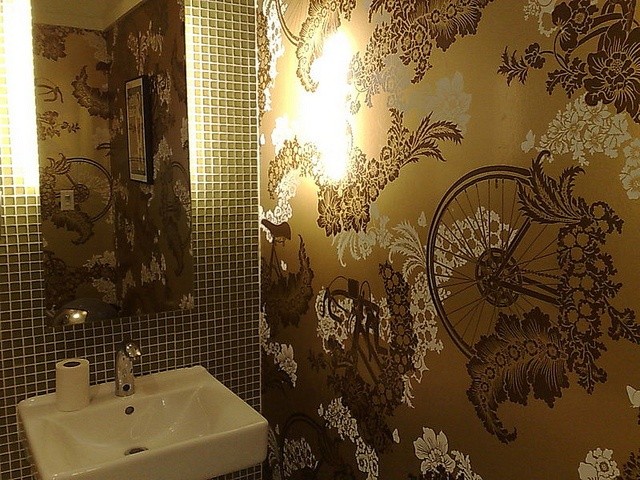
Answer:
[124,75,154,184]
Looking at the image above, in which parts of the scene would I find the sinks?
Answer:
[17,365,269,480]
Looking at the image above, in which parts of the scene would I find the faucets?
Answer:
[50,309,88,327]
[115,341,142,397]
